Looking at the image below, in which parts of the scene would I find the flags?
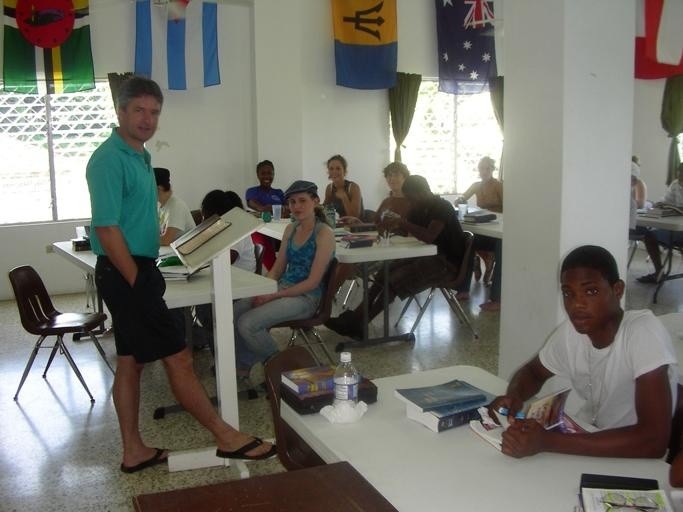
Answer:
[332,0,398,89]
[634,0,683,79]
[436,0,497,94]
[3,0,95,94]
[134,0,221,90]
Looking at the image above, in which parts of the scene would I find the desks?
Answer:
[278,363,682,511]
[48,234,278,421]
[168,206,281,481]
[628,204,682,304]
[254,214,438,353]
[454,207,505,242]
[132,460,398,512]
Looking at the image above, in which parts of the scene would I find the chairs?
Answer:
[261,344,326,472]
[248,243,267,278]
[236,262,353,400]
[394,230,480,343]
[7,264,115,404]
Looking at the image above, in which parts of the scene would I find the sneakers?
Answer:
[324,309,363,342]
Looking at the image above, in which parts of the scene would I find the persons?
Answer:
[454,156,503,282]
[630,155,648,235]
[452,236,502,310]
[669,449,683,488]
[325,175,466,341]
[636,163,683,285]
[86,76,278,472]
[154,167,197,247]
[313,161,409,326]
[191,191,257,375]
[488,245,677,459]
[234,180,336,392]
[313,155,366,222]
[245,160,291,273]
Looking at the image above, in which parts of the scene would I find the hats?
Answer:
[283,180,318,200]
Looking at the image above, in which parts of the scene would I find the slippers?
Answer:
[216,436,277,461]
[120,447,169,474]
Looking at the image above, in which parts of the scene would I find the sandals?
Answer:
[636,272,657,284]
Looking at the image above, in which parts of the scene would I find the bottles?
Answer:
[333,351,360,426]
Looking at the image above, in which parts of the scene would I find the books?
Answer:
[638,204,683,217]
[574,474,675,512]
[344,222,377,233]
[173,214,232,256]
[392,380,486,412]
[339,232,379,249]
[407,380,499,433]
[469,387,590,458]
[462,210,497,227]
[282,364,369,401]
[157,266,189,281]
[71,236,91,251]
